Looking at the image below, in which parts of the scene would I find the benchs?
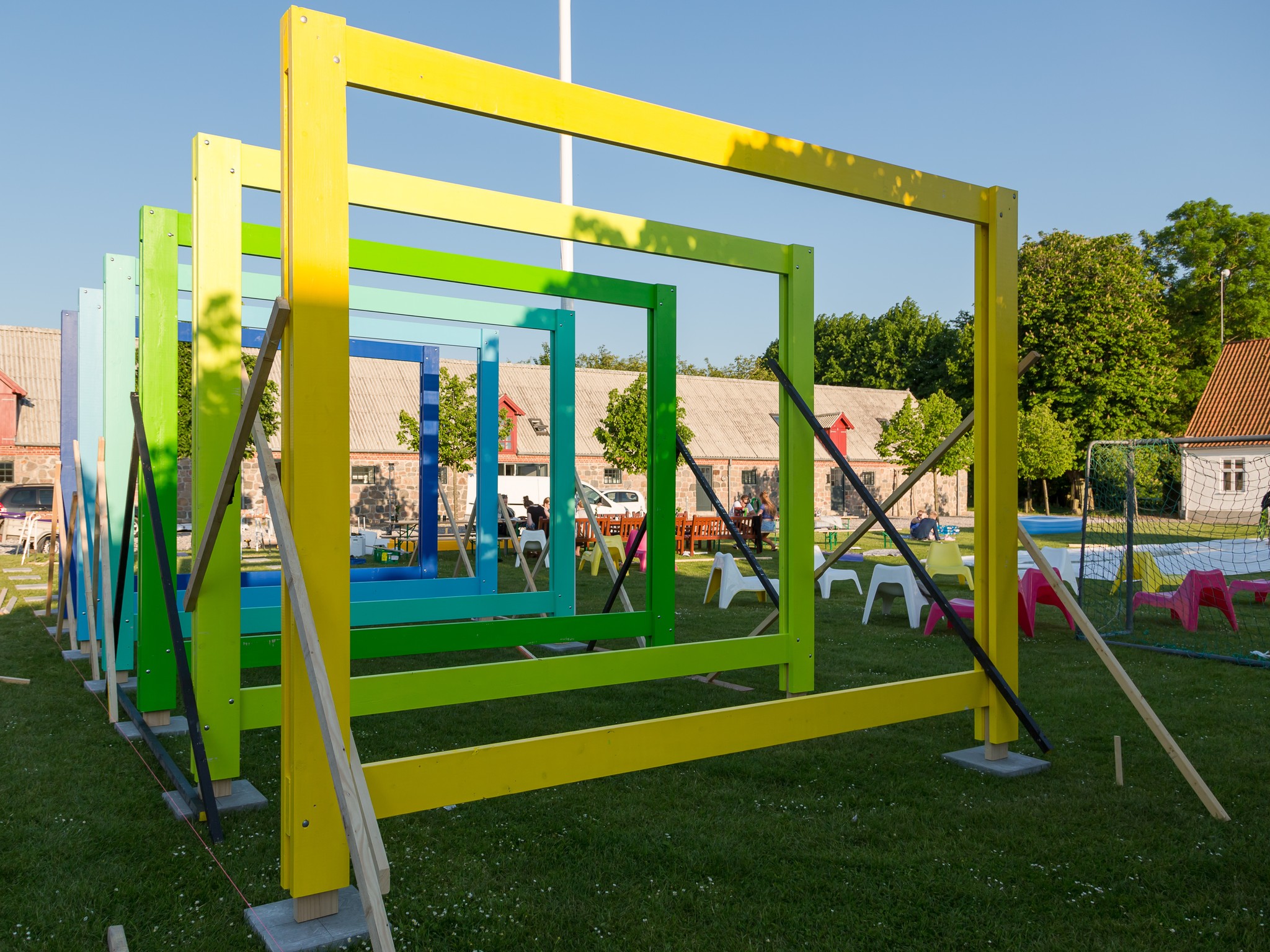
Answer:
[379,510,902,557]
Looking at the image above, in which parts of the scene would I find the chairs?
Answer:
[1124,569,1238,631]
[814,545,862,599]
[1017,546,1079,596]
[1022,564,1076,633]
[1109,548,1187,594]
[1227,578,1270,603]
[862,564,944,628]
[924,572,1035,637]
[624,529,678,572]
[703,552,779,608]
[578,535,630,576]
[924,541,974,591]
[514,529,550,568]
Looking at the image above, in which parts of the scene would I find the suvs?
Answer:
[0,483,140,538]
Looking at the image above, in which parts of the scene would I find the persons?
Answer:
[750,490,779,551]
[497,494,596,556]
[909,510,941,541]
[728,495,757,550]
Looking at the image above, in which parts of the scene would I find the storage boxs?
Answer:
[374,549,398,564]
[350,558,366,566]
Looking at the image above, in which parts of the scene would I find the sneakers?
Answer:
[944,536,955,541]
[732,544,739,549]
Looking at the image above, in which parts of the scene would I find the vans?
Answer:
[465,473,632,530]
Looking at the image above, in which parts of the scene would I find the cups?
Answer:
[498,514,501,520]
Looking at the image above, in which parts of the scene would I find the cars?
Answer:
[580,488,648,517]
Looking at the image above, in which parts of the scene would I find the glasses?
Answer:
[502,495,507,498]
[743,501,749,504]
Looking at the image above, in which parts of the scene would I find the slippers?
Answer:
[771,549,778,551]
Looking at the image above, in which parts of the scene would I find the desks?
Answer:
[711,516,859,551]
[384,518,526,561]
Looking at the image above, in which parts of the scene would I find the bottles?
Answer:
[639,506,644,517]
[615,514,619,520]
[601,514,604,518]
[716,511,720,517]
